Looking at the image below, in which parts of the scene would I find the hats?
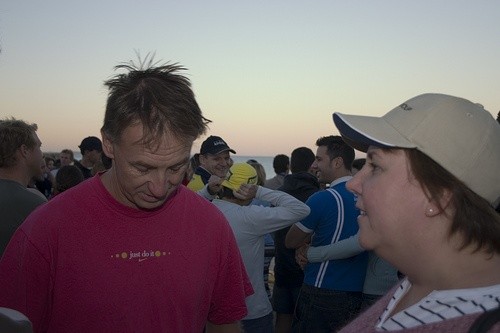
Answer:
[200,135,236,156]
[220,162,258,191]
[332,92,500,211]
[53,165,87,193]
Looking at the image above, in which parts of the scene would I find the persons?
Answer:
[0,116,406,333]
[332,93,500,333]
[0,50,255,333]
